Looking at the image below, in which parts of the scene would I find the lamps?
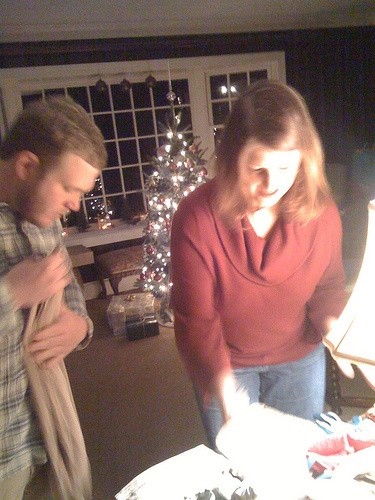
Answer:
[322,198,375,421]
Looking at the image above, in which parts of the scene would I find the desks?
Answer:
[61,219,144,248]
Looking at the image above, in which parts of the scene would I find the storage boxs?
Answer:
[107,293,160,340]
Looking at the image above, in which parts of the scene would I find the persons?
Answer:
[170,80,375,461]
[0,94,109,500]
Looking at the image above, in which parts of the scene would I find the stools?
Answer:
[95,245,144,297]
